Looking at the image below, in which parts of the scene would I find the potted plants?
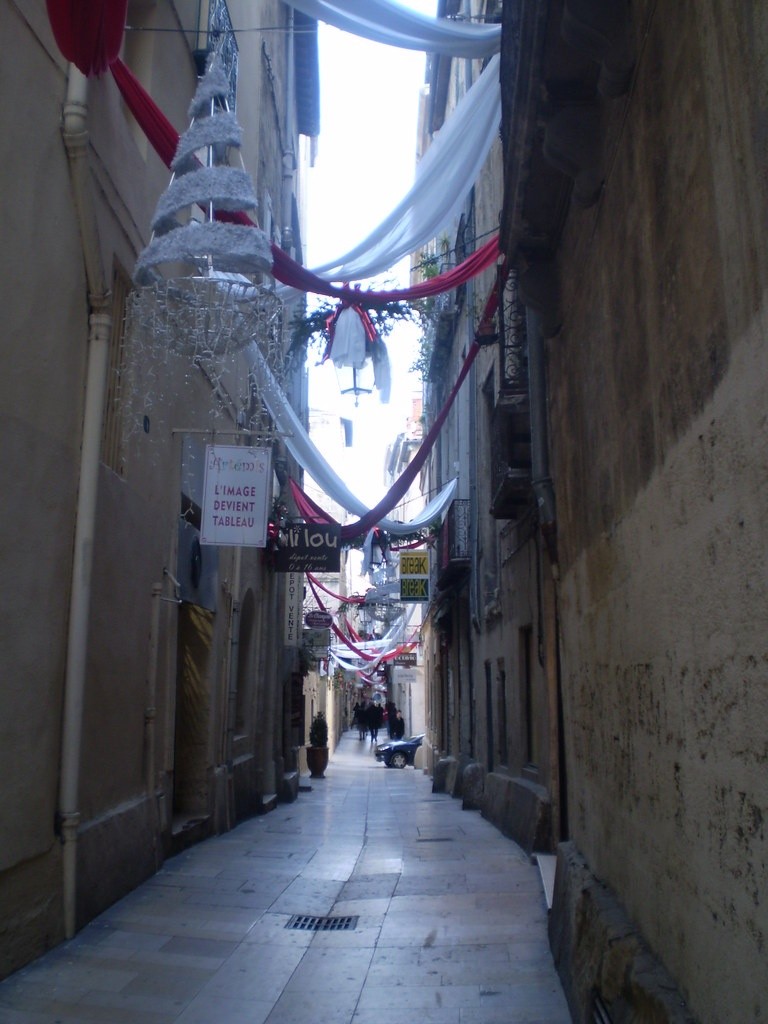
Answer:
[305,711,329,780]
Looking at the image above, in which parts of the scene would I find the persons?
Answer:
[353,700,404,742]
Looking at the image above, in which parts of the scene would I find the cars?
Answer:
[375,734,426,769]
